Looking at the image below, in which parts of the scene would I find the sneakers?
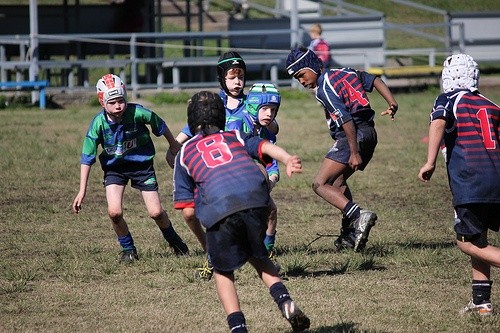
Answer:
[173,241,189,256]
[281,299,310,332]
[268,251,280,273]
[118,249,139,265]
[349,210,378,253]
[459,299,494,317]
[334,232,355,249]
[196,259,214,282]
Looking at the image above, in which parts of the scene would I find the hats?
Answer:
[286,47,322,76]
[217,51,246,98]
[442,54,480,93]
[96,74,127,116]
[246,81,281,126]
[187,91,226,137]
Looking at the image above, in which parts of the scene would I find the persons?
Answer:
[306,24,330,69]
[417,53,500,322]
[285,47,400,252]
[172,91,311,333]
[196,82,282,285]
[166,49,279,167]
[72,73,190,265]
